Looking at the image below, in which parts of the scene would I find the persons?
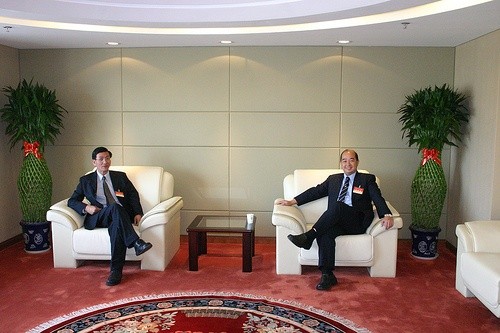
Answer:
[68,147,152,286]
[275,149,395,290]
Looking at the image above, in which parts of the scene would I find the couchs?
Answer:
[456,220,500,319]
[271,170,403,278]
[46,166,184,271]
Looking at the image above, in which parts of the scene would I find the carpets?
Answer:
[24,290,375,333]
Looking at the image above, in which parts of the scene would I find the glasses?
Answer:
[96,157,110,162]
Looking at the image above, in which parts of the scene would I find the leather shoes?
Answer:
[316,272,337,290]
[287,234,313,250]
[134,239,152,256]
[106,272,122,286]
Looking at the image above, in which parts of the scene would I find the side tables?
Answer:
[186,215,256,273]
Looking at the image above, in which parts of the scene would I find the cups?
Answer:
[246,223,254,230]
[246,214,254,223]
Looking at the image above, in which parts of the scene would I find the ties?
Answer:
[102,175,116,205]
[338,177,350,202]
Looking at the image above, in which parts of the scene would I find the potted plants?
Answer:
[0,76,69,255]
[395,84,473,260]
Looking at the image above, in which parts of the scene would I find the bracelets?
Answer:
[384,214,391,217]
